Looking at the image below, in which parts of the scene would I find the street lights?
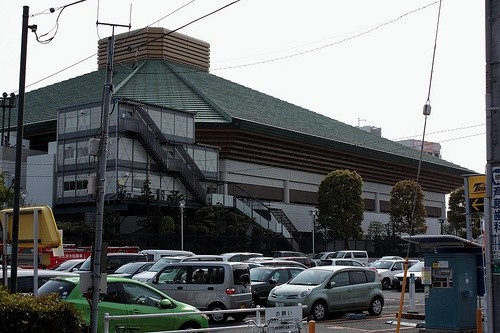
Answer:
[179,199,185,250]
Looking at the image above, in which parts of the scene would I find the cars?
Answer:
[394,262,424,292]
[319,258,366,266]
[52,250,316,283]
[38,276,209,333]
[145,261,252,324]
[267,266,384,321]
[380,256,404,259]
[370,259,420,290]
[0,269,80,297]
[250,267,306,308]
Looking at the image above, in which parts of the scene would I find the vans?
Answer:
[330,251,368,266]
